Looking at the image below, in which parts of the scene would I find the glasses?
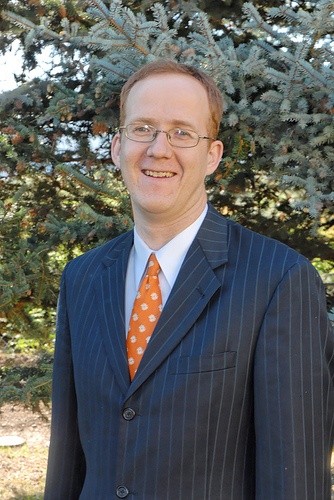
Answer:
[114,123,213,149]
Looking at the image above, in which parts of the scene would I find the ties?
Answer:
[126,254,162,379]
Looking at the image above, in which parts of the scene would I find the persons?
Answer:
[41,59,334,500]
